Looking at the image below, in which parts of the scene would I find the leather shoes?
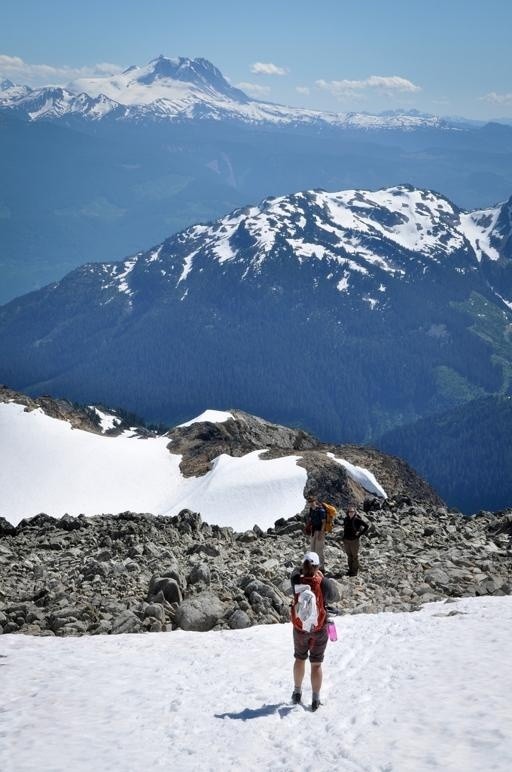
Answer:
[312,700,320,711]
[292,690,302,704]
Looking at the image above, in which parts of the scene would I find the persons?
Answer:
[343,507,369,576]
[306,495,327,575]
[288,551,341,711]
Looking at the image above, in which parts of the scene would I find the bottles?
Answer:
[327,620,337,642]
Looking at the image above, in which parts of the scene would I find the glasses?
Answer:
[308,500,315,503]
[349,510,355,513]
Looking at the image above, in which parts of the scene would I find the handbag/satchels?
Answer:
[304,521,312,536]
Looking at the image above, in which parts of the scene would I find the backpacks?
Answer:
[291,575,326,633]
[322,502,336,532]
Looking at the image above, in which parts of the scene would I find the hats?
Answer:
[303,552,319,565]
